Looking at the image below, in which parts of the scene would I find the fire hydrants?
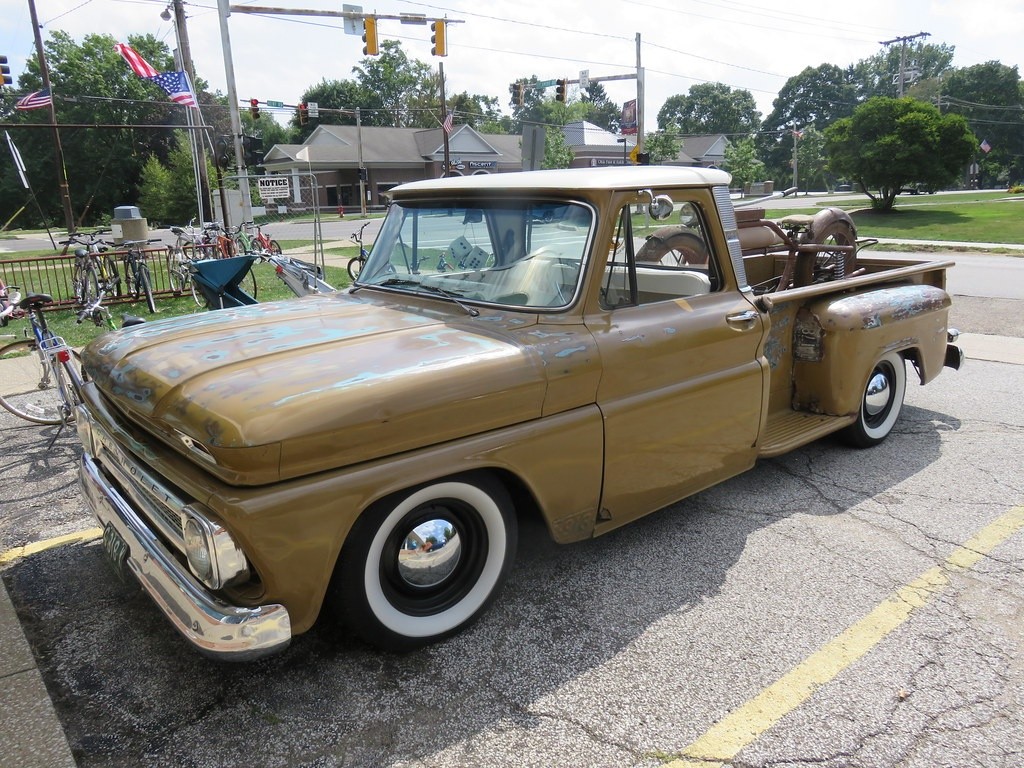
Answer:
[338,204,344,218]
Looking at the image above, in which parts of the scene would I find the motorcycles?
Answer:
[634,186,879,295]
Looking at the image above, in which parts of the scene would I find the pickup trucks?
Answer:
[75,162,964,663]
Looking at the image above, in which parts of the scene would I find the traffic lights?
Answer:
[512,83,524,105]
[241,134,264,165]
[218,138,229,167]
[358,167,367,180]
[556,78,566,102]
[250,98,259,112]
[0,55,12,84]
[299,103,309,125]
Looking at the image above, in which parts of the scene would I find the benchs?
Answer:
[550,262,712,306]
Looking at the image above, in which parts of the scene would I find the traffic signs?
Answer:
[538,79,556,87]
[267,100,283,107]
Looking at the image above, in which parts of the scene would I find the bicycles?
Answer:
[347,222,397,281]
[77,275,146,331]
[167,214,282,308]
[58,228,162,314]
[0,286,83,452]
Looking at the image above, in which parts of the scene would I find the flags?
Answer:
[15,89,51,110]
[443,108,455,133]
[795,128,805,139]
[115,43,196,108]
[980,140,991,152]
